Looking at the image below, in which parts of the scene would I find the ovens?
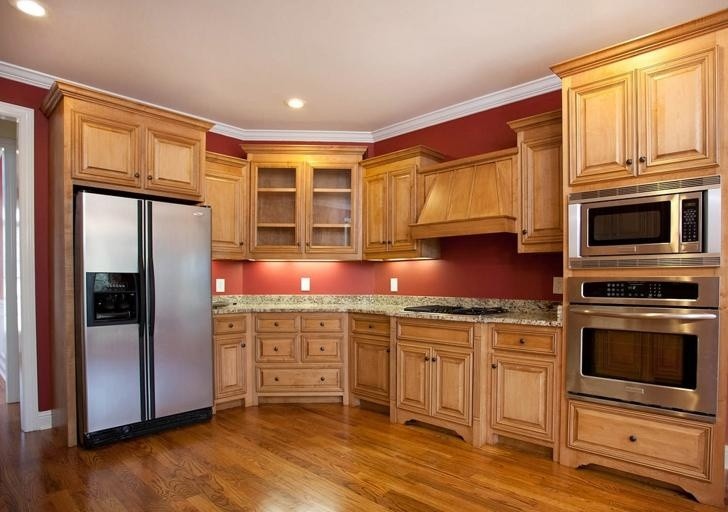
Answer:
[565,275,718,424]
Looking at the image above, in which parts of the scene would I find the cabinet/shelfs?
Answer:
[547,8,728,189]
[563,397,718,484]
[486,322,562,446]
[390,316,486,427]
[348,312,390,408]
[240,143,369,262]
[49,94,217,202]
[254,312,346,398]
[197,151,251,261]
[360,146,447,261]
[212,312,254,401]
[506,109,563,255]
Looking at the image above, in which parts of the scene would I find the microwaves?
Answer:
[581,188,706,258]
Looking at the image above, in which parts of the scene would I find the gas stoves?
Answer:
[405,303,508,317]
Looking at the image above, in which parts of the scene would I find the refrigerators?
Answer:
[72,189,216,449]
